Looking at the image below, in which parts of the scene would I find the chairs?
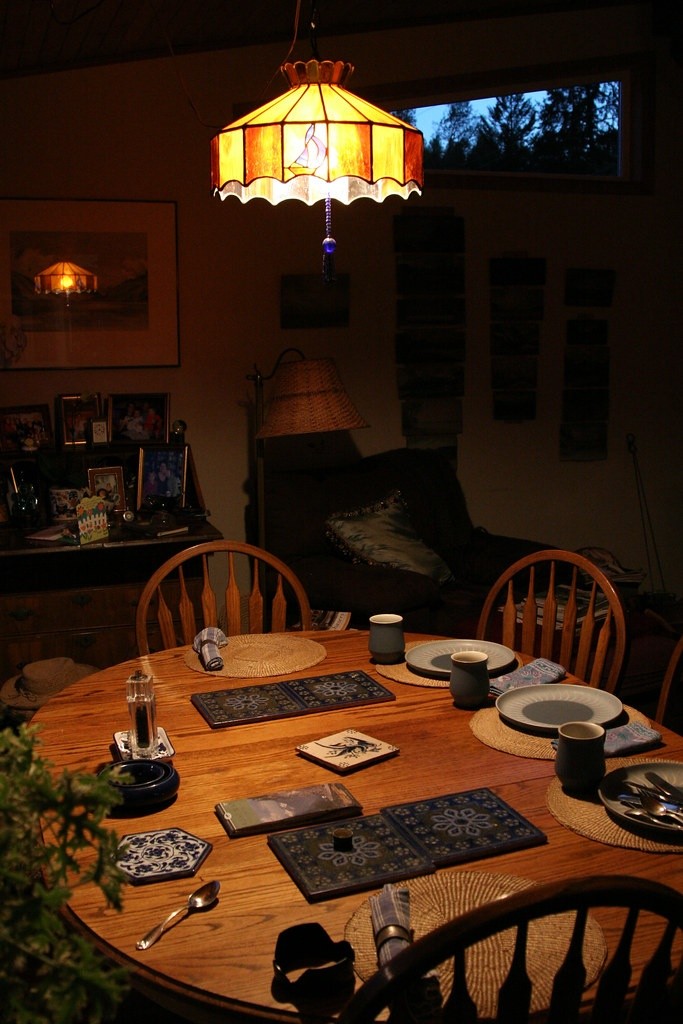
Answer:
[136,540,313,656]
[477,550,628,695]
[270,873,683,1024]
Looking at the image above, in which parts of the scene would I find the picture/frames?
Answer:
[0,196,181,372]
[0,403,54,457]
[87,465,126,512]
[280,273,351,330]
[107,392,171,444]
[137,445,189,514]
[58,392,102,445]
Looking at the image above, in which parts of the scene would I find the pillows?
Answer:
[320,489,456,585]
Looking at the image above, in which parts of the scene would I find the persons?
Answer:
[143,461,180,498]
[403,431,527,605]
[113,402,162,439]
[1,415,42,435]
[94,480,113,500]
[66,411,88,442]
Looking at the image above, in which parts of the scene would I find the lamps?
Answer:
[208,0,425,279]
[34,256,98,308]
[245,346,372,628]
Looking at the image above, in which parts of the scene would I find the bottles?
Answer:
[126,670,160,756]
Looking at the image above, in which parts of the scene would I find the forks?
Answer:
[637,788,683,816]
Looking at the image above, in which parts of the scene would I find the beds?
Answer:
[254,445,683,737]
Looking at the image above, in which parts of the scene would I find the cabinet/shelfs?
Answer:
[0,441,224,691]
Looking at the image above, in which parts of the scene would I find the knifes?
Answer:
[625,782,683,805]
[617,795,683,813]
[644,771,683,802]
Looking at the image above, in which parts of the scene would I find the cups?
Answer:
[368,614,405,664]
[450,651,490,708]
[554,722,607,791]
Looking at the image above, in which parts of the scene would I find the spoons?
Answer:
[136,880,219,952]
[641,797,683,826]
[625,808,683,828]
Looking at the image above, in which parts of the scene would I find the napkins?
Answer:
[193,626,229,670]
[550,722,663,759]
[489,657,566,697]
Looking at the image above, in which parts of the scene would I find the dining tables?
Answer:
[18,630,683,1024]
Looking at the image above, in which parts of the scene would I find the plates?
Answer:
[496,683,624,730]
[404,641,517,677]
[114,726,175,763]
[598,762,683,835]
